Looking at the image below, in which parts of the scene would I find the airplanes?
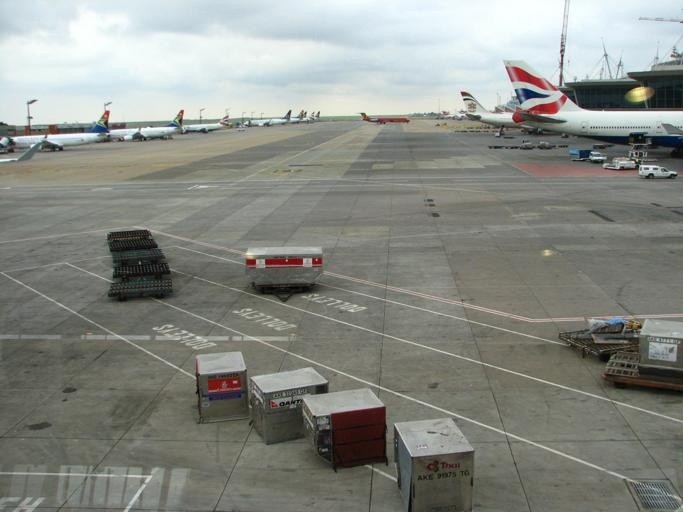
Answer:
[441,57,683,158]
[355,112,411,125]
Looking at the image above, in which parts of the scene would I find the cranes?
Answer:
[638,16,683,23]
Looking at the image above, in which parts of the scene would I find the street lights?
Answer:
[27,99,37,134]
[104,102,112,112]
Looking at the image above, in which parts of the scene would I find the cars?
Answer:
[638,164,679,179]
[589,151,641,170]
